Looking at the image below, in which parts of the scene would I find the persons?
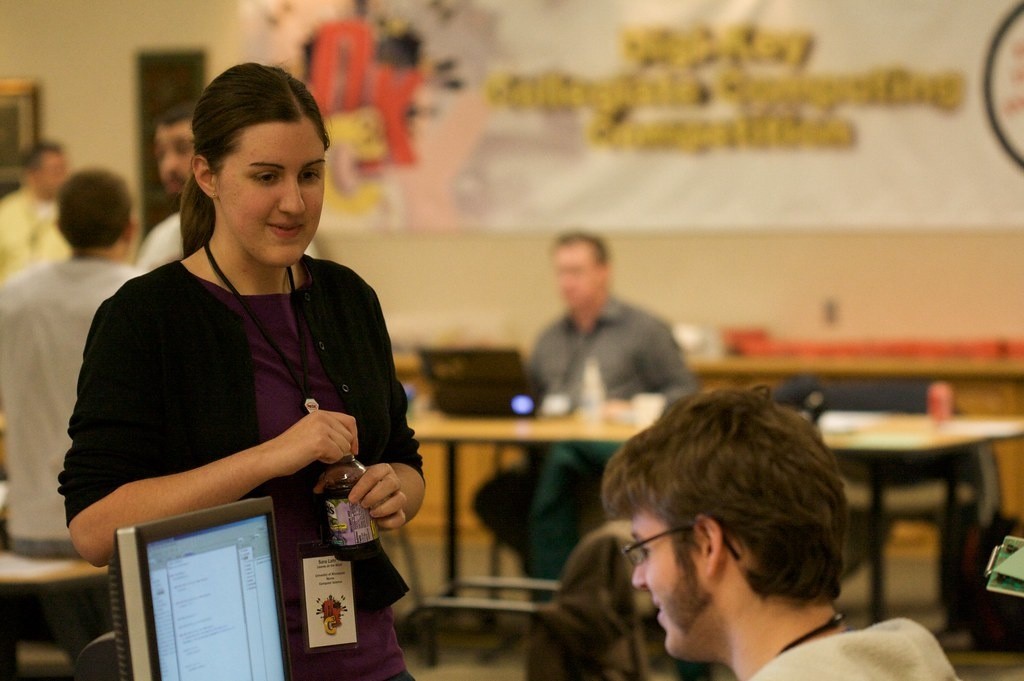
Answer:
[0,102,196,665]
[64,62,425,681]
[602,383,962,681]
[471,232,696,633]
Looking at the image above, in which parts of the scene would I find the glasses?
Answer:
[621,524,740,566]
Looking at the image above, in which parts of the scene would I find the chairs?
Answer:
[779,383,996,625]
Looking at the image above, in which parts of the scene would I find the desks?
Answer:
[393,357,1024,560]
[407,412,1024,666]
[0,552,108,681]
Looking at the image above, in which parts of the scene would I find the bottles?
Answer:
[580,356,605,429]
[323,450,380,562]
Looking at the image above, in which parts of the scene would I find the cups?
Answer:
[633,393,665,427]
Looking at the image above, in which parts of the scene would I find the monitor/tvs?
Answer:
[108,496,294,681]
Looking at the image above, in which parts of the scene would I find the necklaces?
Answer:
[774,613,846,657]
[204,242,320,415]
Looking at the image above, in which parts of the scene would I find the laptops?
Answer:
[415,344,546,416]
